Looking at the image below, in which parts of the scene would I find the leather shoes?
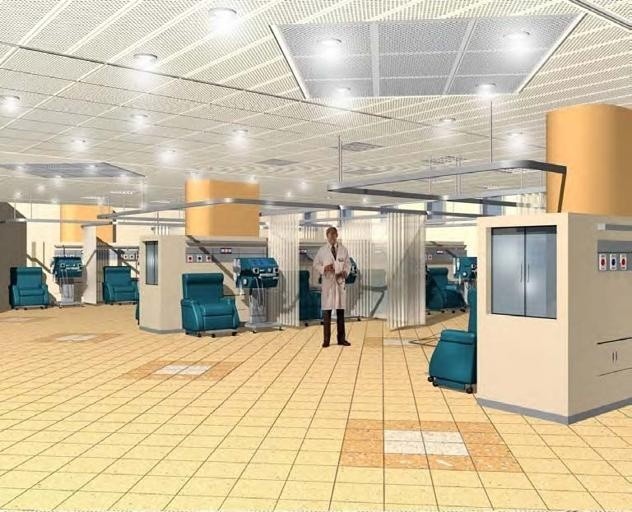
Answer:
[338,340,350,346]
[323,341,329,347]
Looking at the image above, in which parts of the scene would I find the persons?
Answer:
[312,227,351,347]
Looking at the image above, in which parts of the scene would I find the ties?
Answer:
[331,244,336,260]
